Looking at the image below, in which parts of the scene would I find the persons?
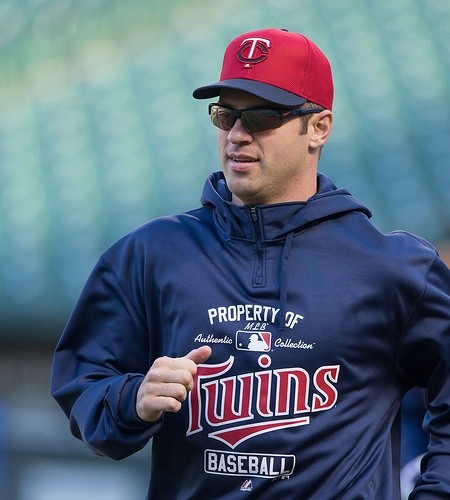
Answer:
[50,28,450,500]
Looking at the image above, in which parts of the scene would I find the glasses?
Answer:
[208,104,321,133]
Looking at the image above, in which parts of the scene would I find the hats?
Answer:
[191,29,333,112]
